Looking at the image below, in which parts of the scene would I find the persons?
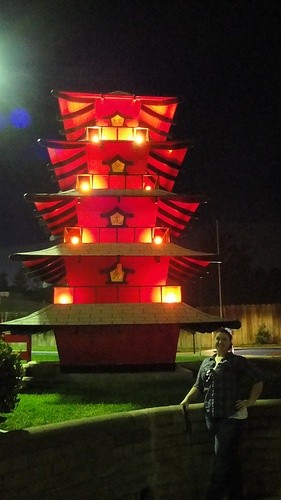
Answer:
[178,328,266,500]
